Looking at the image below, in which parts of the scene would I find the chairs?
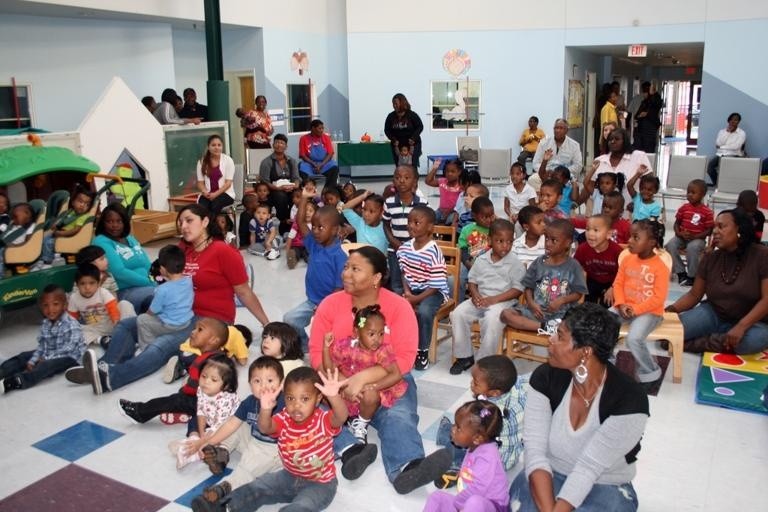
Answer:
[3,190,100,265]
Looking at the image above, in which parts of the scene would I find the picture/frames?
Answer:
[566,78,586,129]
[430,80,481,132]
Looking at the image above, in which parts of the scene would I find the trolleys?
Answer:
[1,144,152,315]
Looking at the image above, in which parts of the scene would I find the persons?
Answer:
[2,80,768,512]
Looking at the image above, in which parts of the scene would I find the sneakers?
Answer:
[117,398,146,425]
[99,336,113,350]
[65,366,89,385]
[413,349,428,370]
[203,443,227,476]
[434,470,462,488]
[287,249,297,270]
[163,354,180,384]
[159,412,192,425]
[352,419,367,444]
[449,354,475,375]
[191,484,228,511]
[226,231,237,243]
[267,246,279,261]
[168,435,201,470]
[393,448,453,493]
[341,442,376,479]
[2,375,20,394]
[83,347,110,395]
[678,271,694,286]
